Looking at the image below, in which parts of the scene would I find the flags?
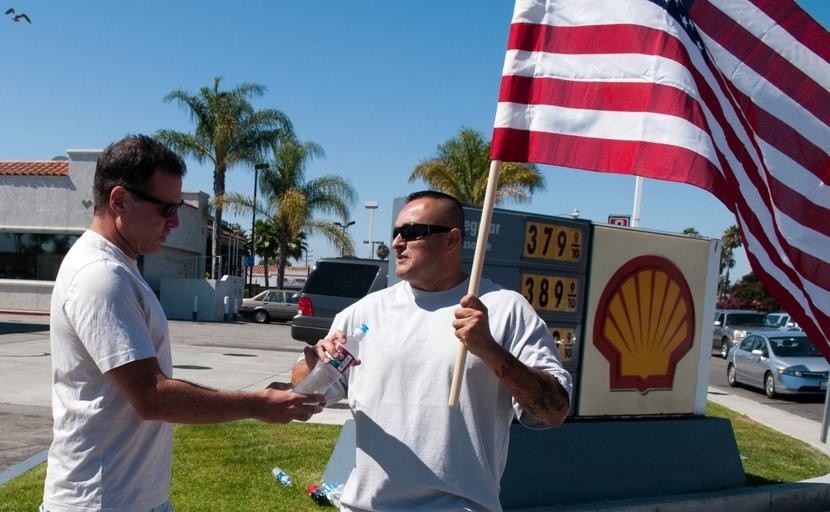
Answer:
[488,2,829,364]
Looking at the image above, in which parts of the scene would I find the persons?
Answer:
[294,190,573,512]
[34,134,327,512]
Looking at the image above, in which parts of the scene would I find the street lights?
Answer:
[365,199,379,258]
[247,161,273,300]
[363,240,384,260]
[556,209,581,218]
[332,220,356,258]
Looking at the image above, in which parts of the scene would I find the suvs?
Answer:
[289,255,389,347]
[710,306,778,360]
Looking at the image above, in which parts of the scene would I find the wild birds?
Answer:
[4,8,31,25]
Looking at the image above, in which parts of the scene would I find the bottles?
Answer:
[305,478,344,507]
[270,464,292,489]
[288,323,369,422]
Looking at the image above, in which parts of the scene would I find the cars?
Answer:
[722,331,829,402]
[239,289,304,324]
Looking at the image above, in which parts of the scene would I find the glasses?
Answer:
[129,190,183,218]
[393,223,454,241]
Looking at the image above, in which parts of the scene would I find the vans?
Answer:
[763,311,805,332]
